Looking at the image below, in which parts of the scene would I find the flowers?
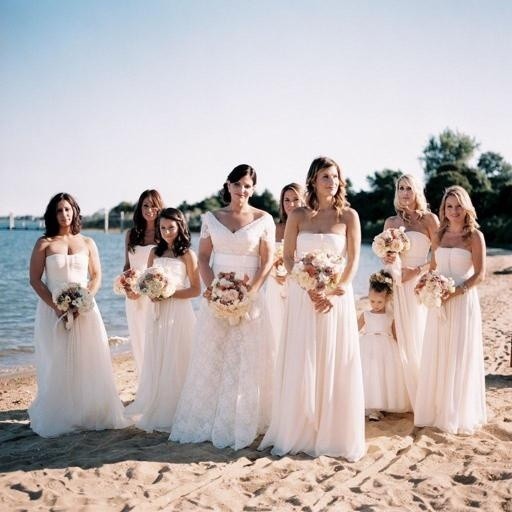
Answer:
[271,249,290,279]
[291,253,349,299]
[371,226,410,269]
[135,267,177,301]
[369,272,393,288]
[111,268,139,302]
[52,281,95,331]
[414,268,457,321]
[202,271,261,324]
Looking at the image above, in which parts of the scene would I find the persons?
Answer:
[273,184,307,286]
[28,194,128,437]
[385,174,441,414]
[126,208,202,436]
[169,164,276,450]
[258,157,368,462]
[114,189,164,369]
[356,273,411,421]
[414,186,489,436]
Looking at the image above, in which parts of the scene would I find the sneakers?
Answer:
[369,412,384,420]
